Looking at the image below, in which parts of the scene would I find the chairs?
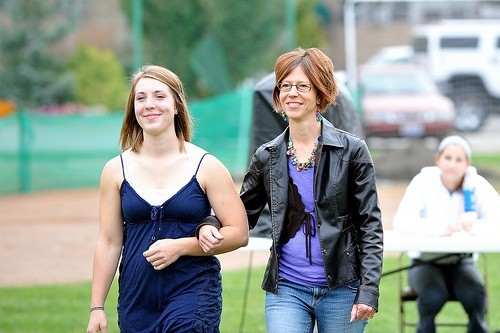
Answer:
[398,251,487,333]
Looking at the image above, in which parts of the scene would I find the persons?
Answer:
[394,135,500,333]
[196,44,384,333]
[87,64,250,333]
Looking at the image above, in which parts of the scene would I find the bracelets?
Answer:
[90,306,104,313]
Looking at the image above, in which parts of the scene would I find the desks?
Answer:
[236,234,499,332]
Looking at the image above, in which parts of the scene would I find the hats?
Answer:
[438,135,471,160]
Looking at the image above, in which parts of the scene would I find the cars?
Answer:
[354,63,455,139]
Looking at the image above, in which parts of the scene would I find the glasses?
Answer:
[277,83,316,92]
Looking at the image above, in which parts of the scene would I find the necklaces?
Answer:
[286,135,321,172]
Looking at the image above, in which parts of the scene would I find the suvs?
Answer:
[412,19,500,132]
